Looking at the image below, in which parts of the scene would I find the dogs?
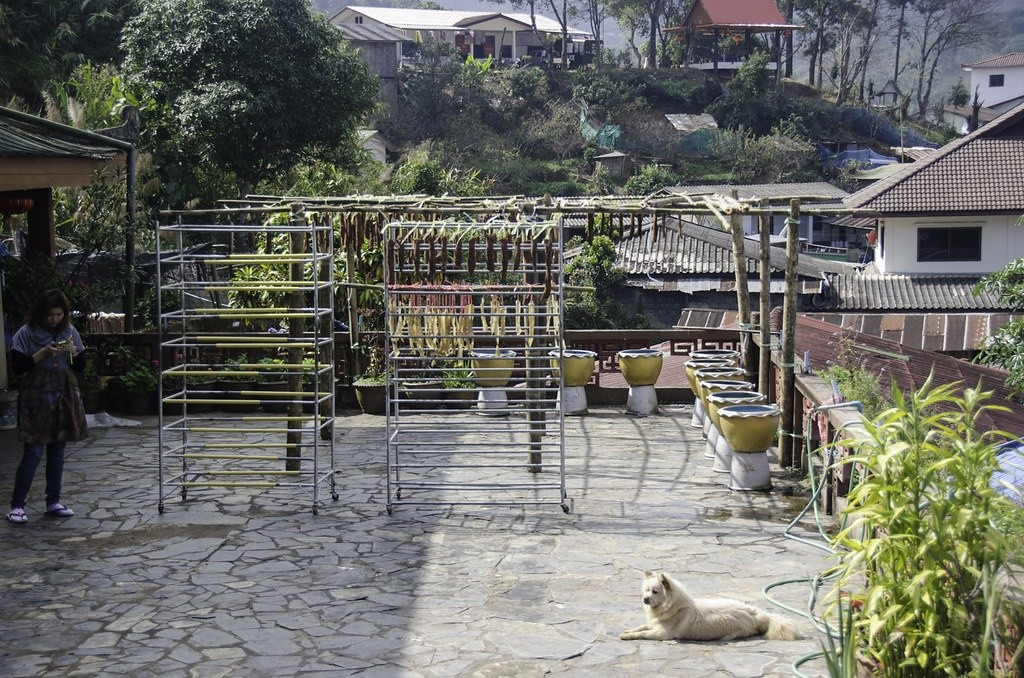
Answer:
[616,566,809,643]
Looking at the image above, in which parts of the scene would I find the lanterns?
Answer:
[733,34,743,46]
[783,30,790,38]
[675,33,684,44]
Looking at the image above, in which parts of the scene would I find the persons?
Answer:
[7,288,91,523]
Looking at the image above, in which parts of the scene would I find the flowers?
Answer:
[152,354,183,389]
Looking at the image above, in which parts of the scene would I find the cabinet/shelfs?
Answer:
[369,218,570,515]
[157,215,339,516]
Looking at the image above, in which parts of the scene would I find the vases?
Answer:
[468,349,517,415]
[684,349,781,491]
[618,349,663,415]
[548,349,597,415]
[161,391,182,415]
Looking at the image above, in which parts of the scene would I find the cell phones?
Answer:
[57,341,66,347]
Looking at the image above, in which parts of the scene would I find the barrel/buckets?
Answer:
[691,397,773,491]
[555,385,589,416]
[624,385,660,416]
[476,388,511,417]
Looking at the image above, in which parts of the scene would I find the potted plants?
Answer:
[181,352,322,413]
[352,308,476,412]
[120,360,157,408]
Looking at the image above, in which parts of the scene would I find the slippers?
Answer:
[6,512,28,524]
[45,505,74,517]
